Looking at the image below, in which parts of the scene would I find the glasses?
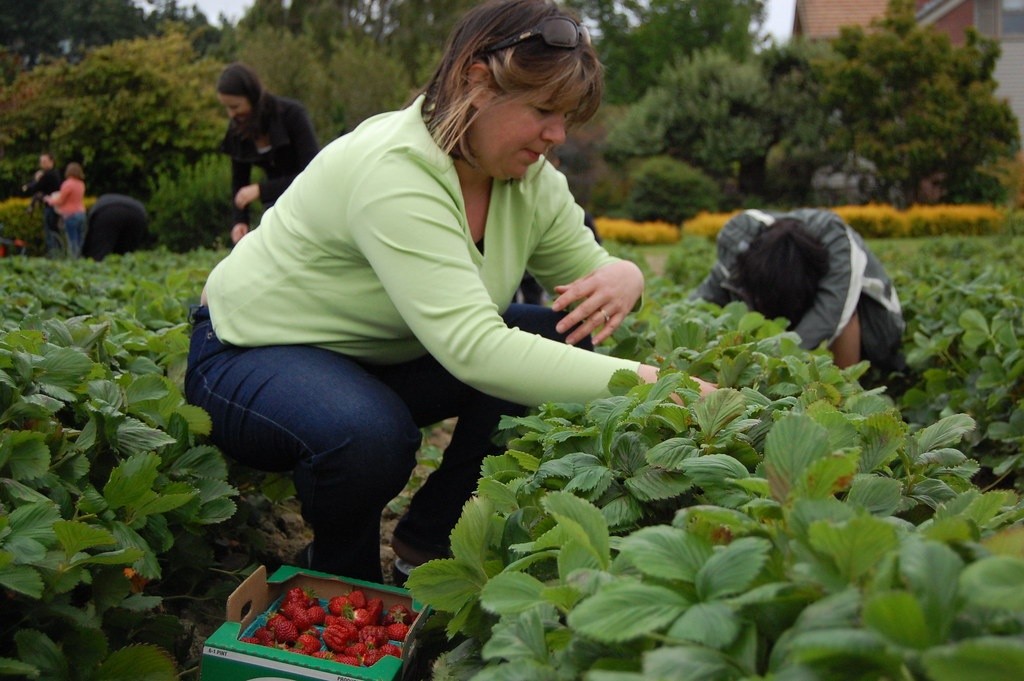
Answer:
[487,17,591,55]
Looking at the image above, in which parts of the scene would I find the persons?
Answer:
[182,2,729,588]
[677,204,903,385]
[42,162,89,259]
[19,150,64,262]
[82,193,153,262]
[212,64,319,246]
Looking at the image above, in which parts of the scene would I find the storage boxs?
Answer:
[197,564,437,681]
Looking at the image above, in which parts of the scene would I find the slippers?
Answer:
[295,543,316,569]
[392,556,433,578]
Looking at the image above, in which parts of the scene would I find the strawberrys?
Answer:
[241,584,422,667]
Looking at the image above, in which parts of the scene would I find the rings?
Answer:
[597,308,610,323]
